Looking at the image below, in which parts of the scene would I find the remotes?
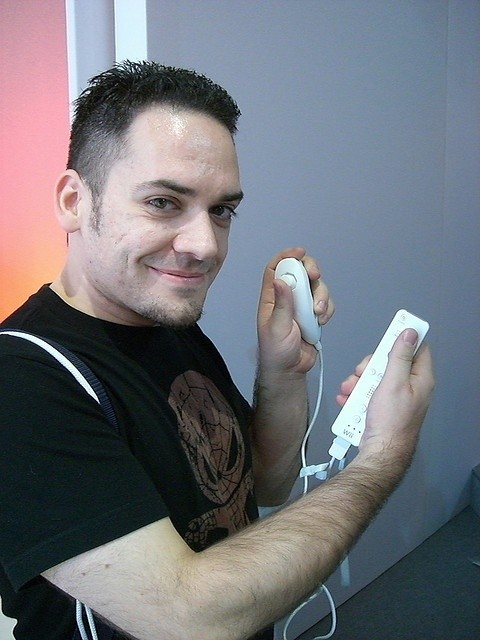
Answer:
[270,258,321,350]
[324,309,436,470]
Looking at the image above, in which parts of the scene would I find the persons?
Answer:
[3,59,436,639]
